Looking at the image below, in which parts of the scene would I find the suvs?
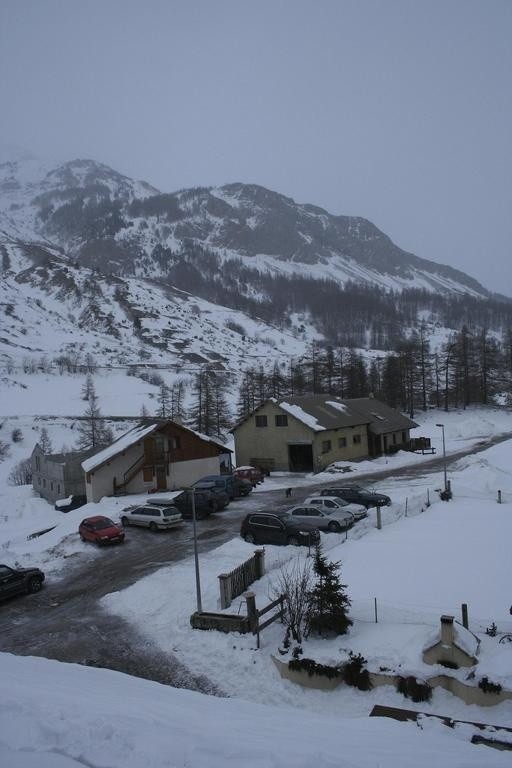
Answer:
[320,484,391,511]
[239,509,321,547]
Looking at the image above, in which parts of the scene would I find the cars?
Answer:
[146,465,264,522]
[283,503,355,533]
[303,495,368,520]
[119,505,184,533]
[0,563,45,604]
[79,515,125,547]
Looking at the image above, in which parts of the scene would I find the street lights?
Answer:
[436,424,447,490]
[180,485,203,616]
